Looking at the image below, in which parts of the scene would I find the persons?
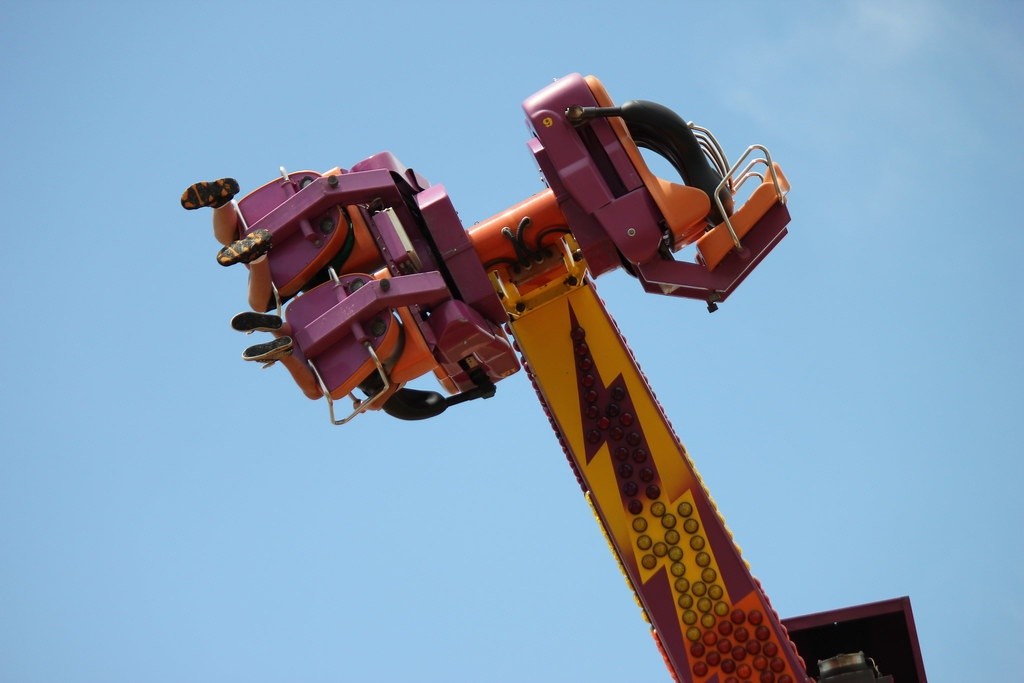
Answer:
[180,178,405,413]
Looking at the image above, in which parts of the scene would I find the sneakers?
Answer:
[179,177,241,210]
[241,335,294,369]
[229,311,283,335]
[216,228,273,267]
